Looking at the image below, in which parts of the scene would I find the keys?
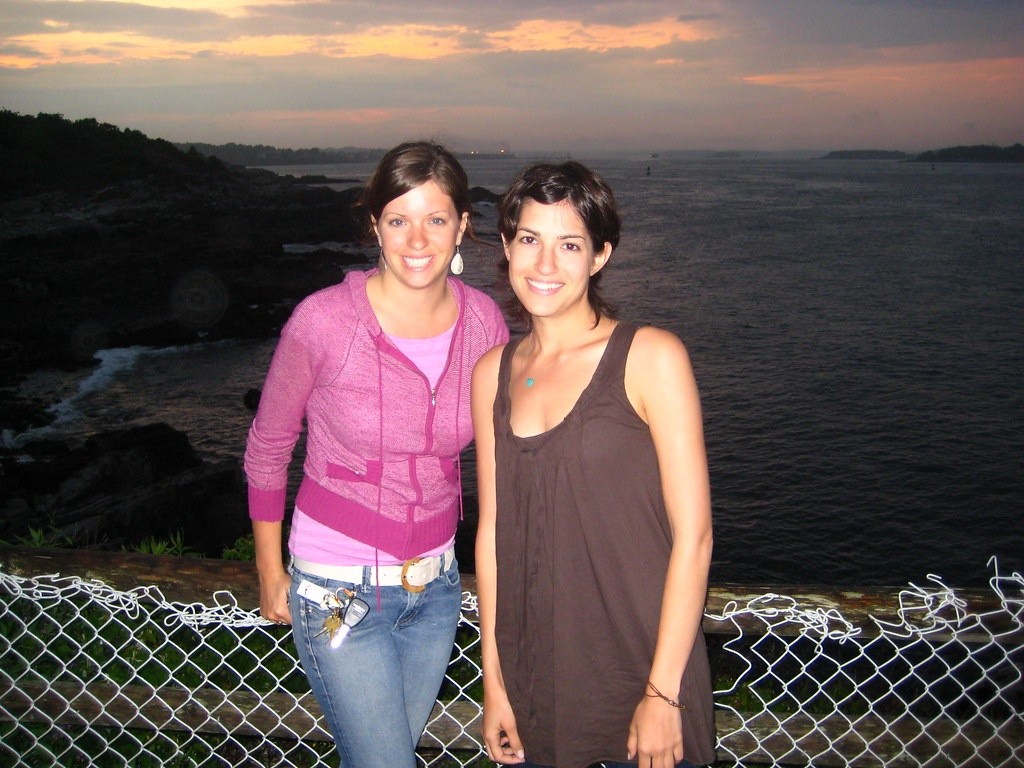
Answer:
[312,595,369,650]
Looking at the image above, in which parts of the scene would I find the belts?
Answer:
[292,546,455,592]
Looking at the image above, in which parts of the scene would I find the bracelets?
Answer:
[643,682,684,710]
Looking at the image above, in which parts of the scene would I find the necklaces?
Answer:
[524,336,584,388]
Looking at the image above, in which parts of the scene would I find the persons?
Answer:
[245,143,514,768]
[433,162,714,768]
